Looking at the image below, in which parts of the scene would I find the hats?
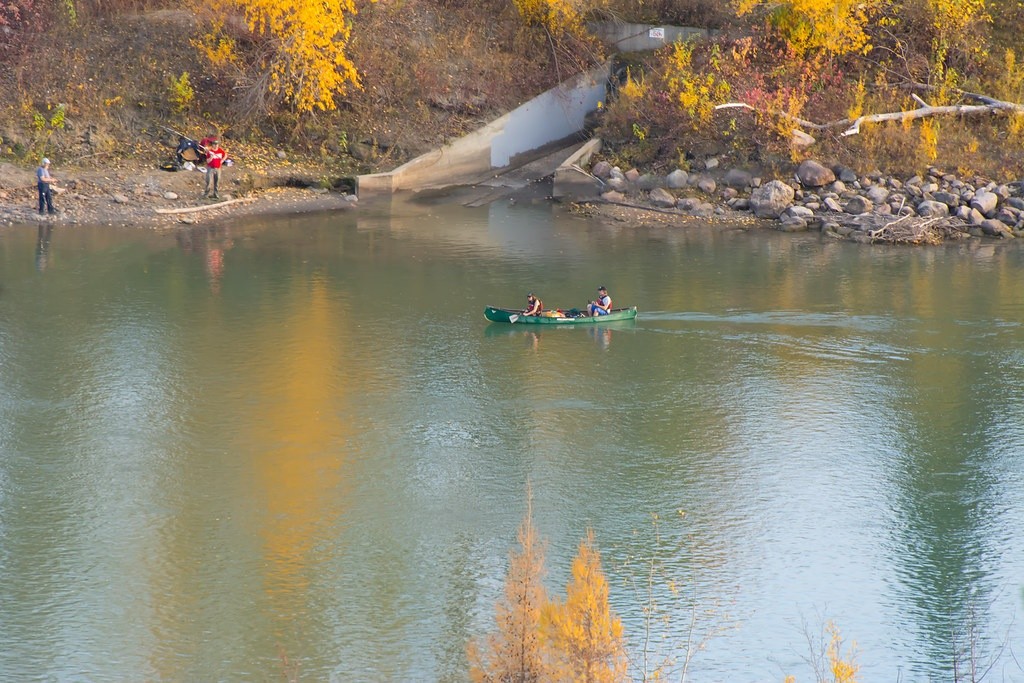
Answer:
[597,286,607,290]
[212,140,219,144]
[526,291,533,296]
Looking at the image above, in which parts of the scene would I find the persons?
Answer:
[587,327,611,353]
[203,139,228,199]
[522,330,541,354]
[36,158,60,216]
[586,286,613,318]
[523,291,543,317]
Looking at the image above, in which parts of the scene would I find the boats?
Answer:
[484,304,637,324]
